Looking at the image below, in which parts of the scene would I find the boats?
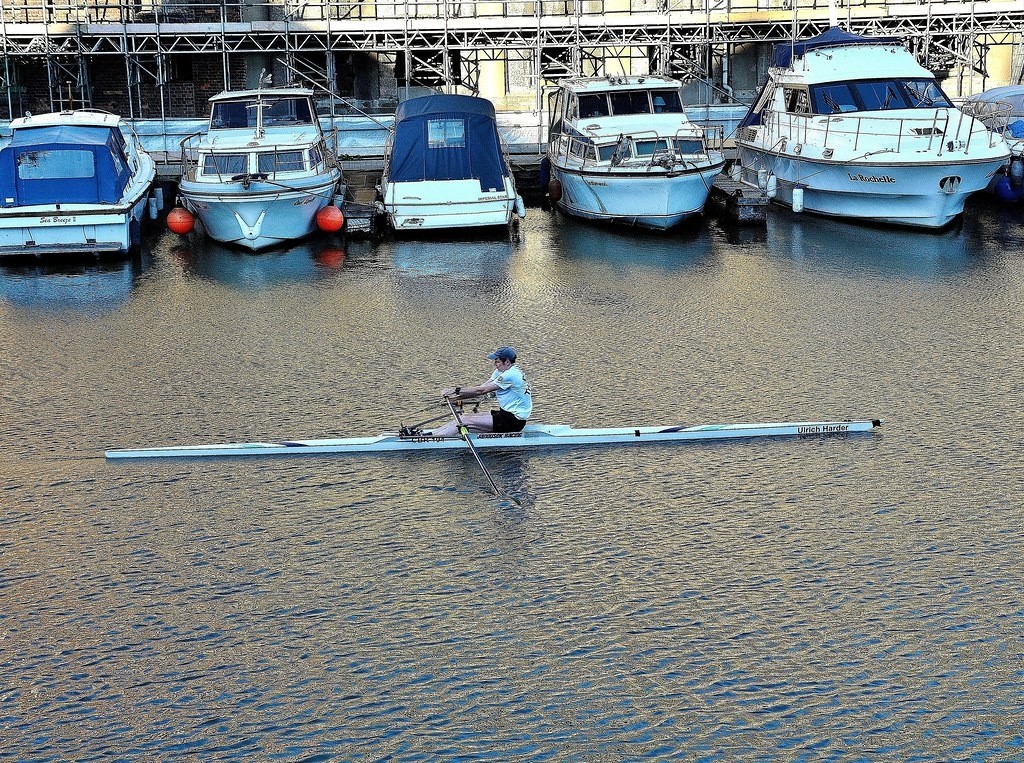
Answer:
[104,418,885,463]
[733,27,1013,229]
[546,74,726,231]
[962,85,1024,157]
[0,109,157,256]
[178,87,347,252]
[375,94,526,230]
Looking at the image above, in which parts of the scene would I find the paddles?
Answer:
[400,390,496,415]
[444,395,504,496]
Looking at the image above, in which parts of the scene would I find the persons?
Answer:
[404,347,533,438]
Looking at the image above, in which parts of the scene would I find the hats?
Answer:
[487,346,515,360]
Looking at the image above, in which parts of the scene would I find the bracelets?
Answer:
[455,387,461,395]
